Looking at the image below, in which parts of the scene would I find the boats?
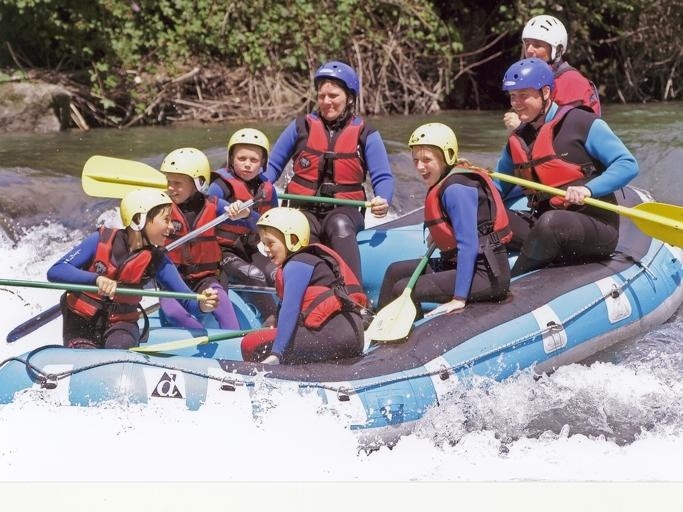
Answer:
[0,182,681,455]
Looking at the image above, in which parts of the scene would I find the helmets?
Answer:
[160,147,209,192]
[227,129,269,171]
[315,62,358,105]
[257,208,309,252]
[120,188,172,231]
[503,58,554,93]
[407,123,457,167]
[522,14,567,60]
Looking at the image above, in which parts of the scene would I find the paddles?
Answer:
[468,165,682,249]
[5,188,265,343]
[81,154,372,207]
[364,240,437,341]
[128,328,272,353]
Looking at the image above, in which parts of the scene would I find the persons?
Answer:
[207,127,285,328]
[377,122,514,324]
[503,14,602,128]
[242,207,366,365]
[158,146,262,328]
[46,190,220,350]
[490,58,640,277]
[263,61,393,291]
